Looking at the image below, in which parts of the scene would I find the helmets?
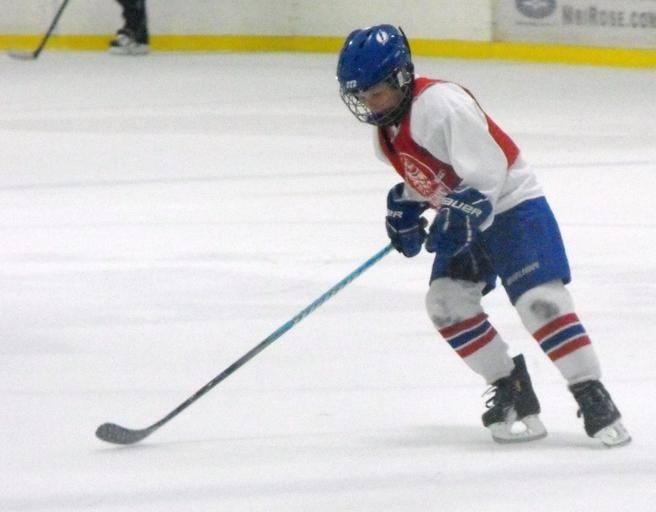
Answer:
[337,25,413,128]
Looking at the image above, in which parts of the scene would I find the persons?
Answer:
[108,1,150,47]
[335,24,621,438]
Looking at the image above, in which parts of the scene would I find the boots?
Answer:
[570,381,621,438]
[484,352,540,430]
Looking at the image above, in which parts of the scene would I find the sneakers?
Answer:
[112,28,136,46]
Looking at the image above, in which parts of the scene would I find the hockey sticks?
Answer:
[7,0,68,59]
[95,217,428,444]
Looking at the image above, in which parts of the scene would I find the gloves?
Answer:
[429,187,491,257]
[384,180,429,258]
